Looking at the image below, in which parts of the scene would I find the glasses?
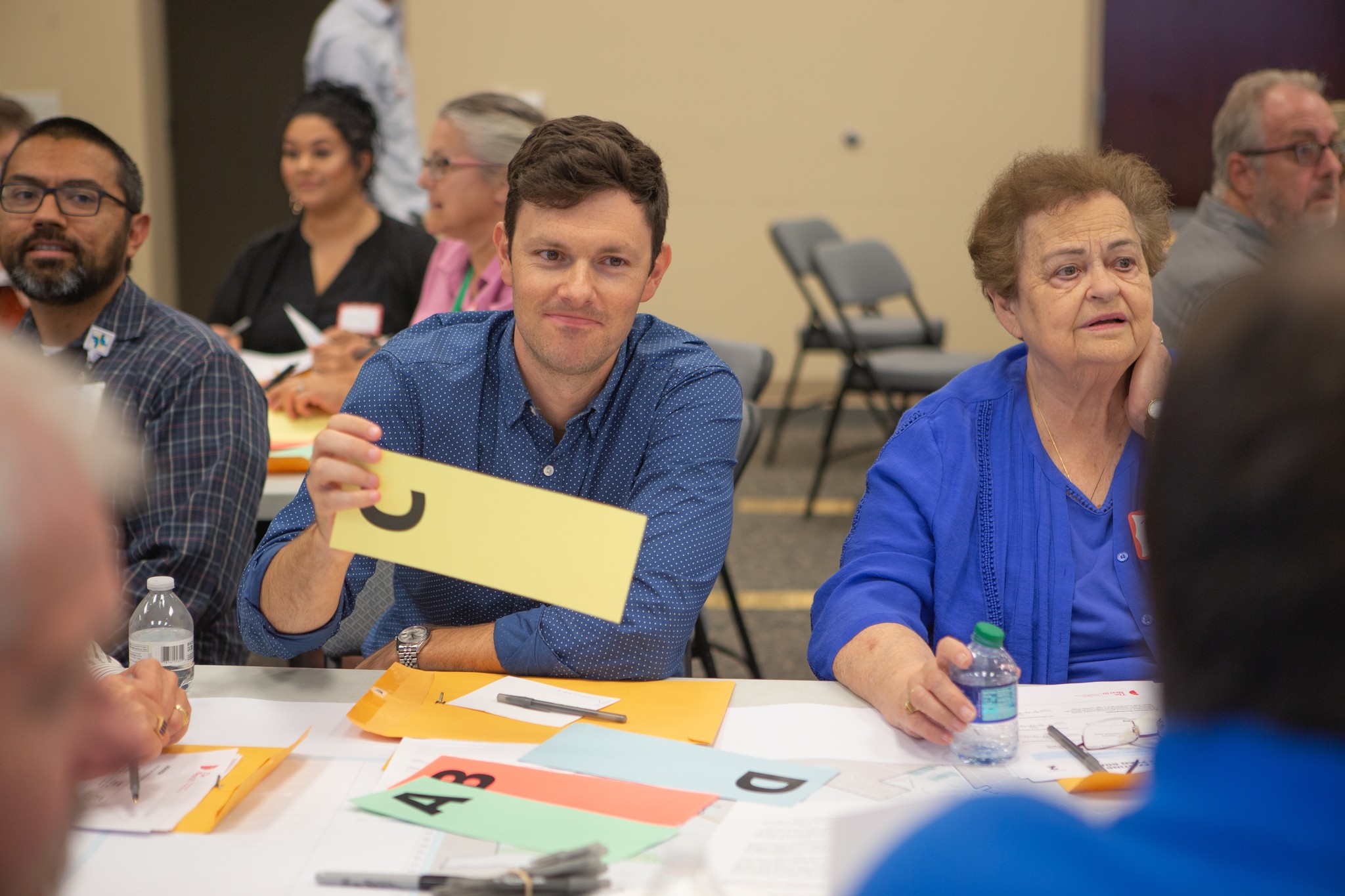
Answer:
[422,151,510,174]
[1076,716,1163,783]
[1242,129,1345,165]
[1,177,133,217]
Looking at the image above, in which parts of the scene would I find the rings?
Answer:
[173,705,190,727]
[1160,338,1164,343]
[156,714,169,741]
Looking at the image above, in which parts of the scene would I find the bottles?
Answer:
[947,622,1019,766]
[128,576,195,696]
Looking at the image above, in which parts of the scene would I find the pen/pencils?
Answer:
[1047,726,1109,773]
[497,694,627,723]
[265,363,297,390]
[432,844,611,896]
[226,316,251,339]
[129,674,139,804]
[317,872,465,889]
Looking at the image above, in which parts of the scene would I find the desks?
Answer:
[58,665,1163,896]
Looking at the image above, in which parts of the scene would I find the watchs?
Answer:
[1143,398,1169,448]
[394,622,441,671]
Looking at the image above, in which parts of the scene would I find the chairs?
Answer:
[682,221,991,677]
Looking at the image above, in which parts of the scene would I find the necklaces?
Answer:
[1027,371,1127,501]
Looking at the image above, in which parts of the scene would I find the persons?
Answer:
[303,0,430,227]
[1154,68,1345,384]
[0,115,272,678]
[0,95,50,327]
[233,114,745,681]
[0,325,191,896]
[843,231,1345,896]
[265,93,549,421]
[205,80,436,358]
[807,145,1192,747]
